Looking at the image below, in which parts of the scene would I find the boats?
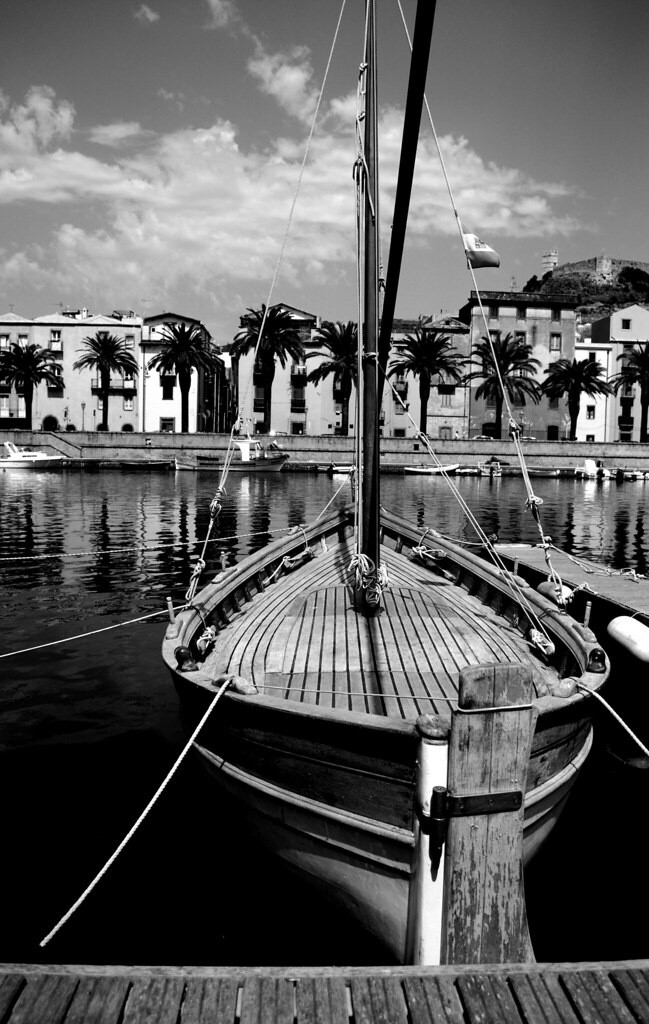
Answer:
[0,441,66,467]
[404,463,460,474]
[528,470,562,477]
[318,465,356,473]
[176,433,291,472]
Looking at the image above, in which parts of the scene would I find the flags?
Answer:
[457,216,501,270]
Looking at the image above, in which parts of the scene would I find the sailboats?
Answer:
[153,2,613,789]
[187,728,599,870]
